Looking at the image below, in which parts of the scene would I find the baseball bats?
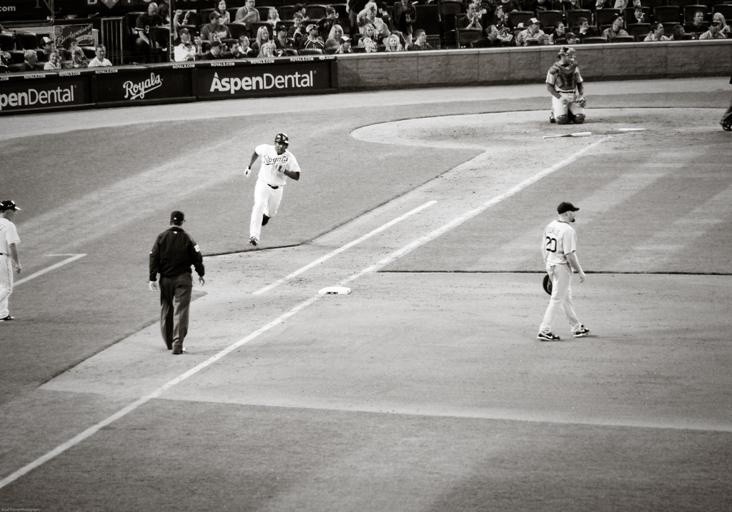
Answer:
[605,127,647,135]
[543,132,593,139]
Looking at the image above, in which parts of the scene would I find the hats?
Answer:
[170,210,184,222]
[557,202,579,215]
[0,200,20,211]
[305,24,319,33]
[528,18,540,25]
[339,36,352,44]
[178,28,191,35]
[209,12,222,19]
[38,37,53,44]
[273,133,288,145]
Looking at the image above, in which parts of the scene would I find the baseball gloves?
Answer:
[543,274,553,295]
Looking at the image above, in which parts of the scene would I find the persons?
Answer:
[536,201,590,341]
[545,47,586,125]
[243,132,301,247]
[0,199,22,321]
[719,75,732,131]
[148,210,206,354]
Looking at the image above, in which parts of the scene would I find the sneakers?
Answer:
[0,315,14,321]
[572,328,589,337]
[536,332,560,341]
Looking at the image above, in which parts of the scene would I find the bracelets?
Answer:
[280,166,286,173]
[248,165,251,170]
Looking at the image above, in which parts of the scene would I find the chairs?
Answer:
[1,30,99,73]
[127,0,732,60]
